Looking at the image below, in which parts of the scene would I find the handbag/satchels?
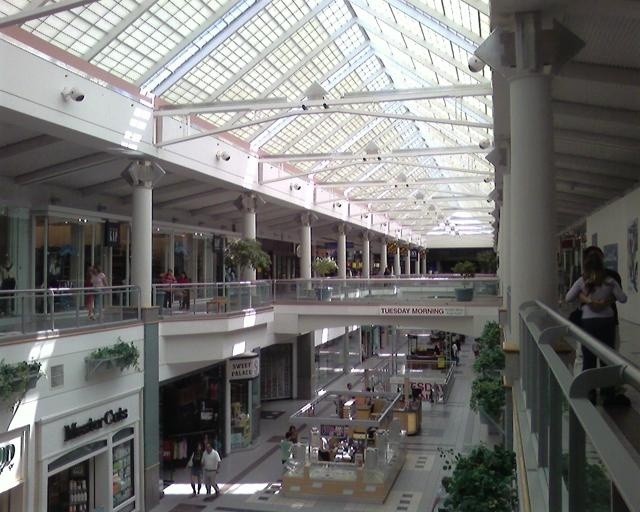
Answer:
[567,307,583,327]
[187,450,195,468]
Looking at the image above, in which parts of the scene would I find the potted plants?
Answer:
[310,254,340,303]
[220,237,273,312]
[449,258,479,302]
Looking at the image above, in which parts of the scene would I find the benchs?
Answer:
[204,294,228,314]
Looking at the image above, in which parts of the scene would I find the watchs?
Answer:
[200,441,222,502]
[318,433,331,462]
[186,443,204,495]
[288,425,298,444]
[279,432,294,464]
[586,296,593,308]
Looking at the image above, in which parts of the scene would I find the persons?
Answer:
[161,267,191,313]
[384,266,390,274]
[48,257,62,275]
[578,246,631,406]
[85,264,108,323]
[335,383,372,415]
[564,248,627,407]
[1,256,17,317]
